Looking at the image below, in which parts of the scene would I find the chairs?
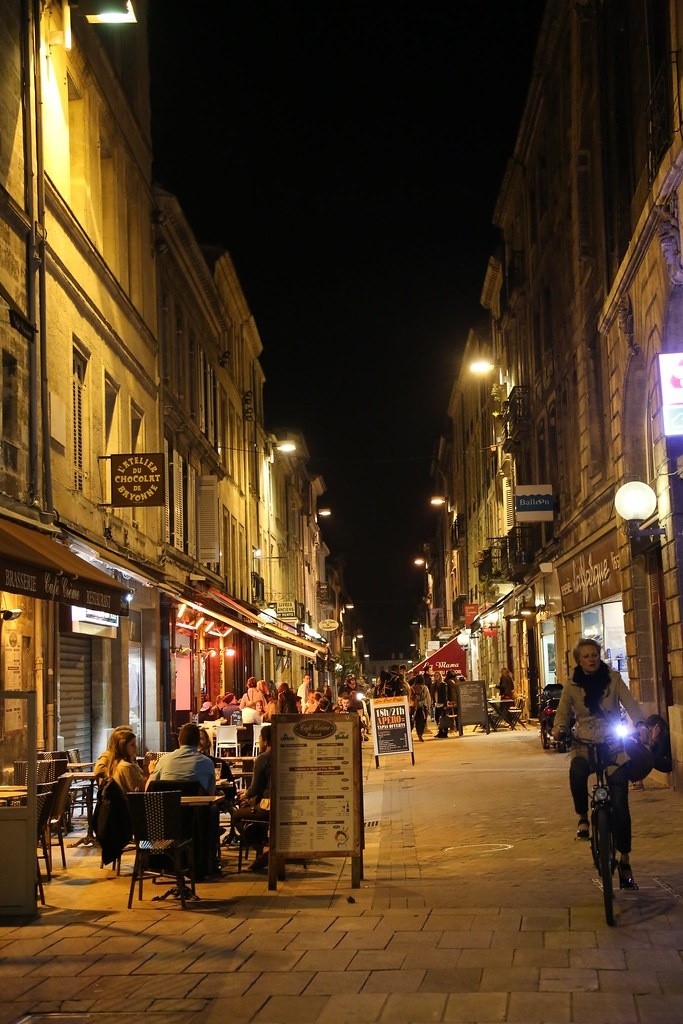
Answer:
[0,723,270,910]
[472,692,527,732]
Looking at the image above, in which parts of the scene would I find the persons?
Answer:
[92,724,133,836]
[199,662,466,741]
[495,668,515,723]
[554,639,650,888]
[145,723,215,795]
[232,726,272,846]
[198,729,236,836]
[108,730,157,795]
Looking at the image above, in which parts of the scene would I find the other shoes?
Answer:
[418,737,424,742]
[628,783,645,792]
[438,734,448,738]
[617,860,634,886]
[576,819,590,836]
[433,732,442,737]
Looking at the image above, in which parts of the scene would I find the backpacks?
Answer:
[411,685,426,709]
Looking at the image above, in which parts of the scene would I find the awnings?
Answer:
[1,515,132,619]
[173,583,329,660]
[60,528,160,588]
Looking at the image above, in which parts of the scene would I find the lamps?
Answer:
[170,644,235,659]
[470,358,501,374]
[520,604,550,616]
[503,614,524,623]
[614,480,666,560]
[272,440,299,453]
[317,508,332,517]
[430,496,449,504]
[252,545,263,559]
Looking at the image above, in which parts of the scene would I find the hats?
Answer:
[222,694,234,704]
[247,676,257,685]
[199,702,212,711]
[345,674,356,680]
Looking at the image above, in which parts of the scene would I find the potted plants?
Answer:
[491,411,502,421]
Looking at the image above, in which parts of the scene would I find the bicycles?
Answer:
[550,730,641,925]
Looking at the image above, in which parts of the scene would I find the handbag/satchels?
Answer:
[438,710,453,730]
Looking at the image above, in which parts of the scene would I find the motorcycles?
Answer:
[536,684,576,753]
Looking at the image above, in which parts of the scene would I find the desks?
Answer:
[487,700,516,732]
[67,763,95,815]
[178,795,224,874]
[0,791,25,807]
[232,772,254,788]
[62,772,99,825]
[221,756,256,789]
[0,786,27,791]
